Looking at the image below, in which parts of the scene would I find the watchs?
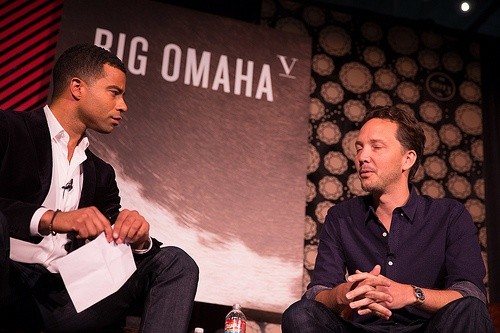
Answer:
[410,285,425,306]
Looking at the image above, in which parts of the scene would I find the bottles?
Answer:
[194,327,204,333]
[224,303,246,333]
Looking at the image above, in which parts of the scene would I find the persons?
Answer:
[0,45,200,332]
[282,104,491,333]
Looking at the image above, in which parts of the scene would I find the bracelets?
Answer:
[48,208,62,236]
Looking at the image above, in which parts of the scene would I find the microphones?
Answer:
[387,252,394,257]
[62,178,73,191]
